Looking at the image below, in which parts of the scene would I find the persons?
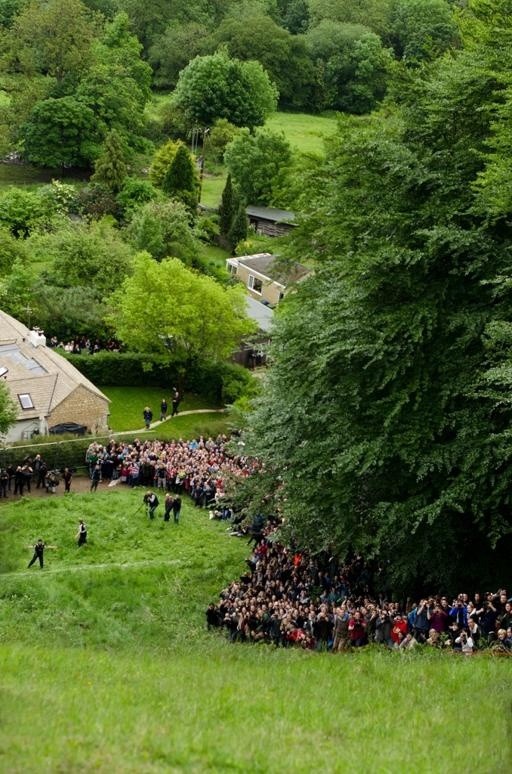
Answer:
[27,539,44,568]
[1,434,211,524]
[47,333,124,355]
[171,392,180,416]
[143,407,153,430]
[205,435,512,657]
[160,398,167,421]
[77,518,87,547]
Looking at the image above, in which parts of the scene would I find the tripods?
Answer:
[132,503,152,520]
